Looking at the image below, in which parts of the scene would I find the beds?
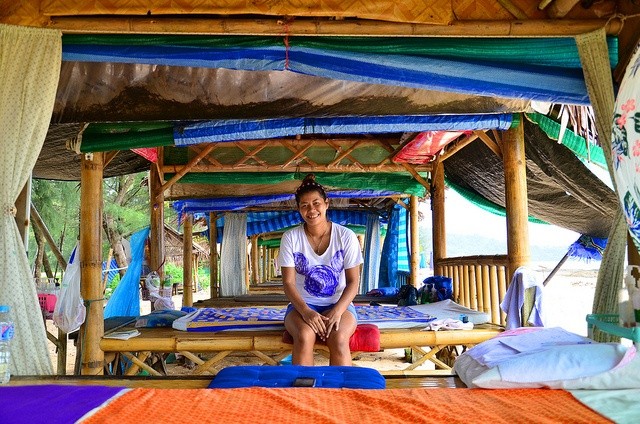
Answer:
[65,112,529,375]
[172,194,419,307]
[248,283,284,295]
[244,234,364,291]
[263,246,283,280]
[0,0,639,424]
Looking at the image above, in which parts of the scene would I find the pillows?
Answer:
[367,287,399,297]
[207,364,386,389]
[136,309,187,328]
[452,327,624,387]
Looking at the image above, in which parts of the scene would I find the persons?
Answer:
[278,173,364,366]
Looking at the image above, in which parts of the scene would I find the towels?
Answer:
[499,266,544,329]
[104,328,142,343]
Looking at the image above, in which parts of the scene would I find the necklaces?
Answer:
[304,221,329,253]
